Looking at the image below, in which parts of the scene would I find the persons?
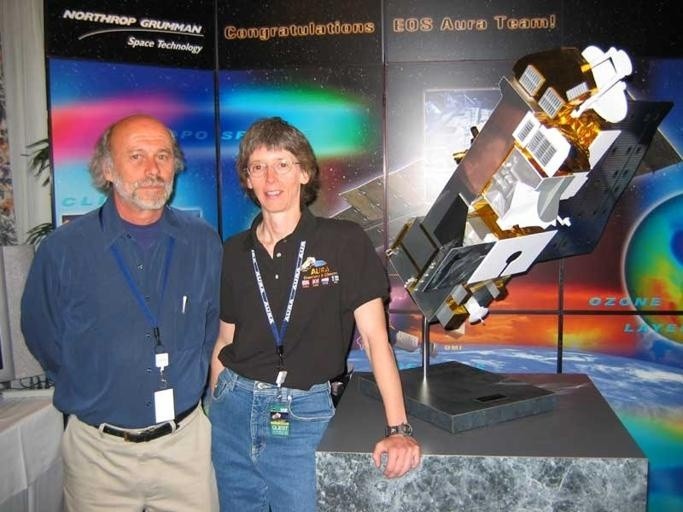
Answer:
[20,113,222,512]
[204,117,422,512]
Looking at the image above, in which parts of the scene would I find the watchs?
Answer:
[385,423,413,436]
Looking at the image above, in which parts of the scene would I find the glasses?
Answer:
[242,159,301,179]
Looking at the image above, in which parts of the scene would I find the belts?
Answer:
[76,400,200,442]
